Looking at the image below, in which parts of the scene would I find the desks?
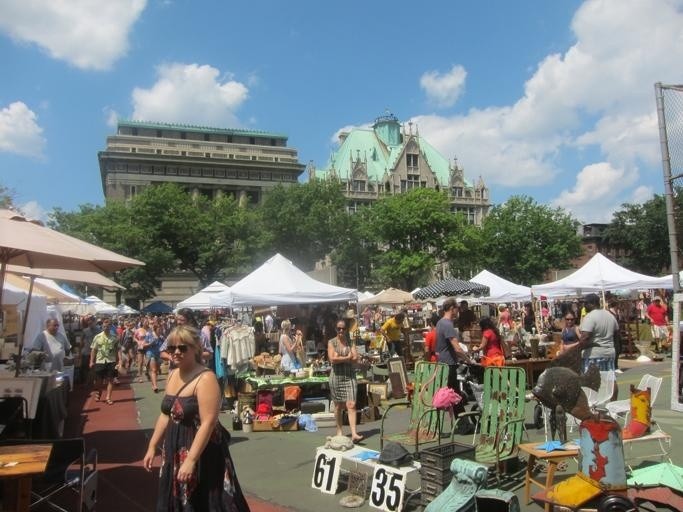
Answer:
[236,362,366,391]
[514,440,579,512]
[0,445,56,512]
[3,371,69,441]
[313,442,420,502]
[502,356,551,391]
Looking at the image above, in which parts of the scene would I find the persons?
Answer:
[140,322,252,511]
[28,288,671,443]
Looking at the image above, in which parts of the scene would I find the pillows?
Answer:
[620,382,651,438]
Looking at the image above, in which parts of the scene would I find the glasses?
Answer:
[335,326,347,332]
[565,317,575,322]
[48,323,60,327]
[167,343,188,354]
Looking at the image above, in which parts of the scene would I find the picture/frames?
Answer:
[387,357,410,399]
[367,382,388,401]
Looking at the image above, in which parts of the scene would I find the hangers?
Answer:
[214,319,249,334]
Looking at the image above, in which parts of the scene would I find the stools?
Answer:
[615,420,672,477]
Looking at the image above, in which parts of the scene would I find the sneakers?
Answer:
[351,434,365,444]
[152,382,160,392]
[95,390,115,406]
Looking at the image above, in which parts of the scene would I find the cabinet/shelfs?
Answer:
[418,440,474,512]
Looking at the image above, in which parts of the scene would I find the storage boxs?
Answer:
[301,394,330,413]
[310,410,338,429]
[254,411,299,431]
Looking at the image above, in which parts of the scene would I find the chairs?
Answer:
[445,363,533,490]
[605,372,662,427]
[38,385,68,435]
[0,396,31,439]
[305,340,317,360]
[569,366,616,434]
[377,357,452,459]
[1,430,100,512]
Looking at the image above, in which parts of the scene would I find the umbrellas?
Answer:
[0,261,128,376]
[411,276,490,309]
[0,203,147,384]
